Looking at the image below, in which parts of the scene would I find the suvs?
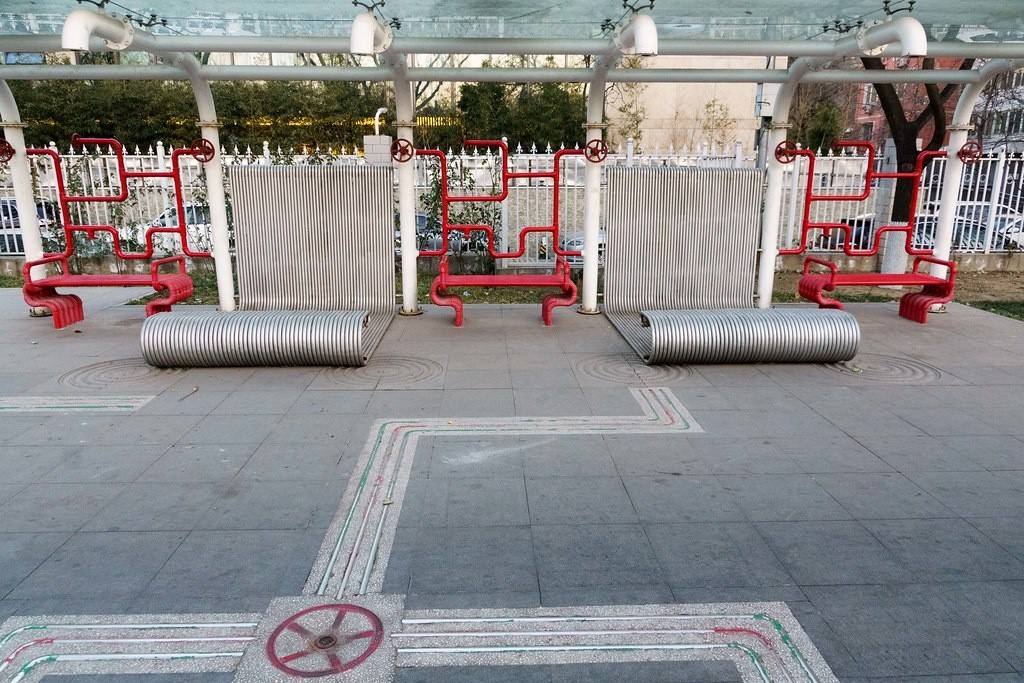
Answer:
[0,196,90,242]
[811,213,1024,252]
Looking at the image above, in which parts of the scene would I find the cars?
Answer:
[108,202,236,255]
[978,214,1024,248]
[393,214,510,254]
[560,227,611,263]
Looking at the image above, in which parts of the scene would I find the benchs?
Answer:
[23,255,195,329]
[431,255,578,326]
[799,256,958,324]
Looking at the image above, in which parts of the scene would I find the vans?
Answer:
[922,201,1022,223]
